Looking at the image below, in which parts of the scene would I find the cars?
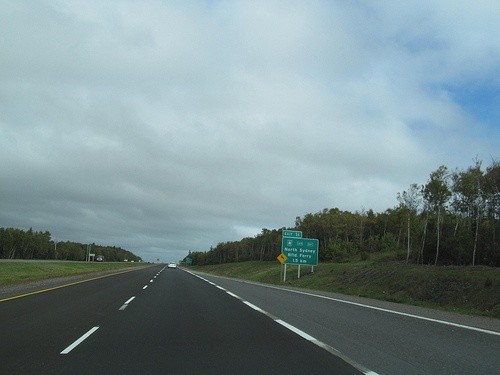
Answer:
[168,263,177,269]
[95,255,142,263]
